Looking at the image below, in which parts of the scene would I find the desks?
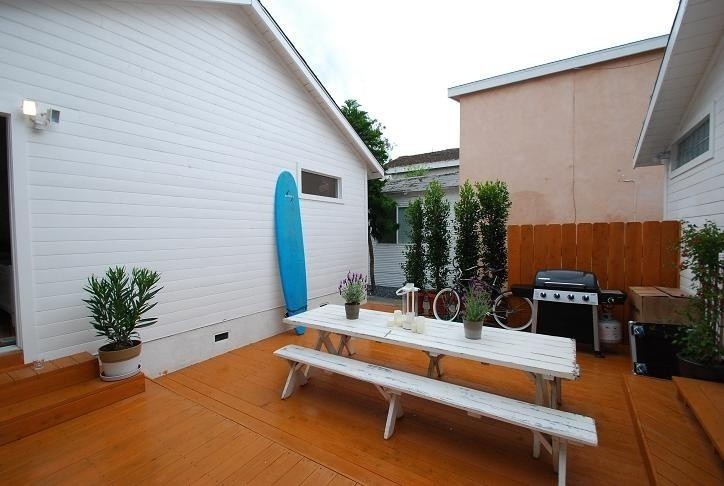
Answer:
[285,301,581,458]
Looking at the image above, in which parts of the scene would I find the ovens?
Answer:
[510,267,627,359]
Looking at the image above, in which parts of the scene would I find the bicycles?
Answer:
[433,255,533,331]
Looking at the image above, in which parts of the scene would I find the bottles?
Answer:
[395,283,421,318]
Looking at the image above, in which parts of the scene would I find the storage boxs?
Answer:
[626,284,706,328]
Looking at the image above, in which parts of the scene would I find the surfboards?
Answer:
[274,170,308,335]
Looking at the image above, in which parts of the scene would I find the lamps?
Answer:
[19,97,63,132]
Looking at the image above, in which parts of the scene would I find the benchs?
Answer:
[271,342,598,485]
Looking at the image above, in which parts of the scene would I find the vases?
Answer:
[462,319,484,339]
[345,303,360,319]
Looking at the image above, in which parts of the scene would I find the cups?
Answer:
[393,311,426,335]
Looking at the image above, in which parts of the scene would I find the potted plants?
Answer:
[336,271,370,304]
[400,177,519,325]
[667,213,724,379]
[81,264,164,380]
